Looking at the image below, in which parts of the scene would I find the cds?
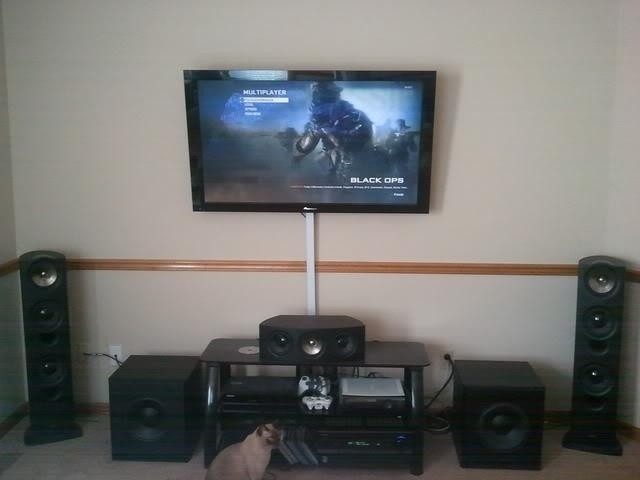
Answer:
[238,346,259,354]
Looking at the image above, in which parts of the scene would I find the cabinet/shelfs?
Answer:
[201,336,430,476]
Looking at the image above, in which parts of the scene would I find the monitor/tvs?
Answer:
[184,70,436,213]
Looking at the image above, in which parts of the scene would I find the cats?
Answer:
[204,419,285,480]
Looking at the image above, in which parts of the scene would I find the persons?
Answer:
[384,119,419,182]
[295,80,374,200]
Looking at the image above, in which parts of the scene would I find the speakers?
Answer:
[18,251,82,444]
[452,359,545,471]
[108,355,201,464]
[561,256,625,457]
[259,314,366,366]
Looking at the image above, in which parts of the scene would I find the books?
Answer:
[273,424,319,465]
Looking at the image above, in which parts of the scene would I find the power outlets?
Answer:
[438,347,455,366]
[108,343,123,365]
